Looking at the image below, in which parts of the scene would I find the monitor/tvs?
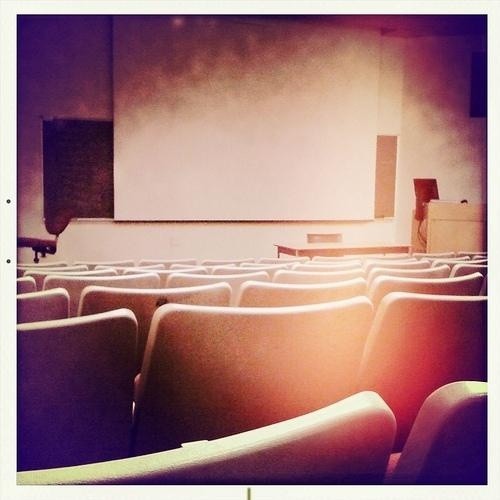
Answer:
[414,178,439,200]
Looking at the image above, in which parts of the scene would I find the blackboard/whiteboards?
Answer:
[42,118,400,223]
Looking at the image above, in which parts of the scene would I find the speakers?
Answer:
[468,51,487,116]
